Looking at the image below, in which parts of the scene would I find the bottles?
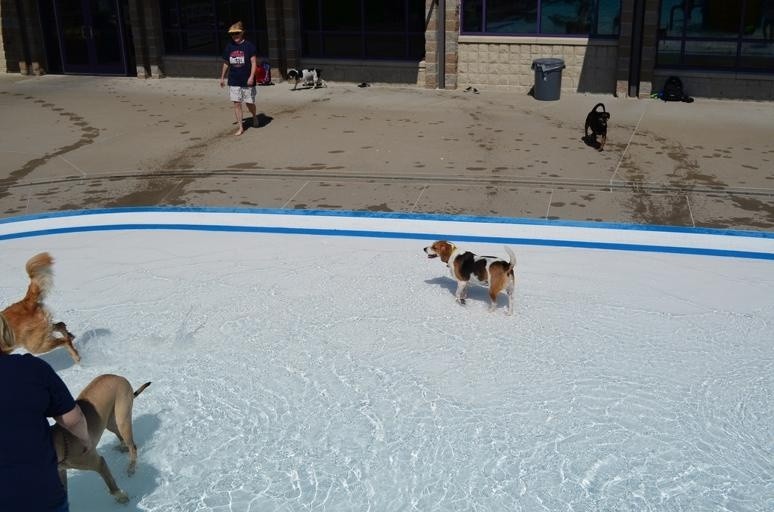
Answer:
[228,27,244,33]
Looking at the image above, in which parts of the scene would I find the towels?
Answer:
[532,58,565,100]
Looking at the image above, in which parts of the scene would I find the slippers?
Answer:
[660,76,694,102]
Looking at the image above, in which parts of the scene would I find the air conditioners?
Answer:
[0,249,80,366]
[423,239,517,316]
[584,103,610,152]
[48,371,153,504]
[285,66,328,91]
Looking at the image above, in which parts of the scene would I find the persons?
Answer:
[218,19,259,137]
[0,313,95,511]
